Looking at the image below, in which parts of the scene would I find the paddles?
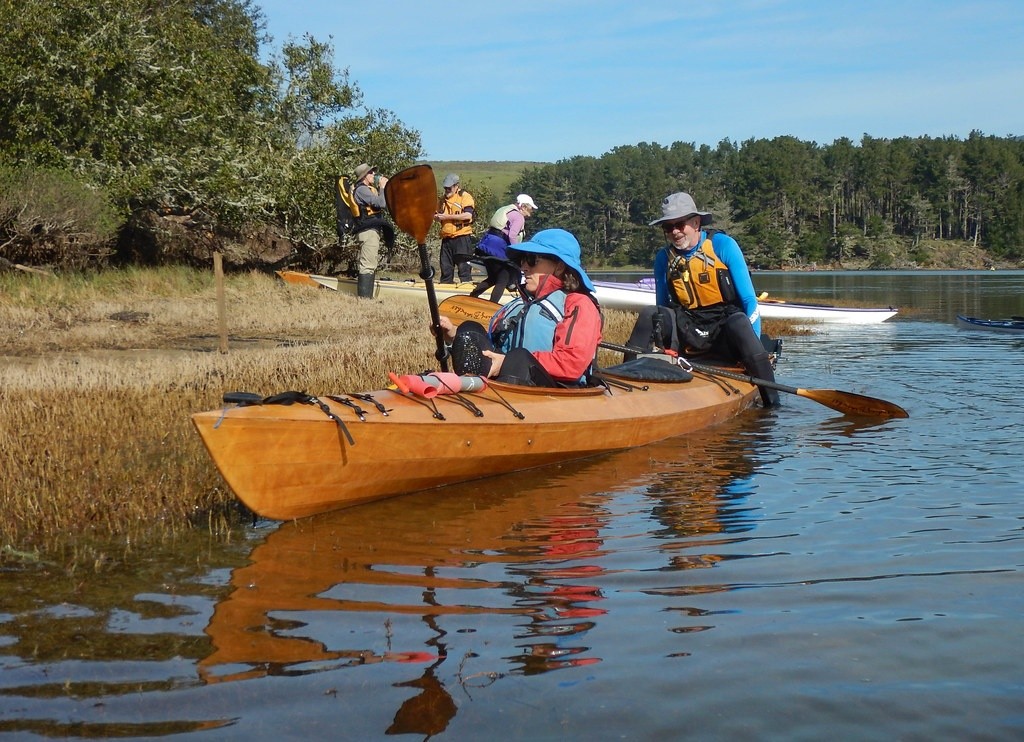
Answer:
[382,162,449,374]
[437,295,912,419]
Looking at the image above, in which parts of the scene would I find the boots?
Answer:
[624,341,648,364]
[747,353,781,408]
[358,274,376,299]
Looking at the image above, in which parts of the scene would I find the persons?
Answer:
[470,193,538,303]
[433,173,475,283]
[348,163,394,300]
[431,229,604,388]
[623,192,780,406]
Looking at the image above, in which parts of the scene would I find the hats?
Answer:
[353,162,378,183]
[648,192,713,226]
[506,229,595,294]
[518,193,540,210]
[441,173,460,188]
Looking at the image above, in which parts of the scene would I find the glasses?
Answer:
[369,171,374,175]
[518,252,560,267]
[662,213,698,234]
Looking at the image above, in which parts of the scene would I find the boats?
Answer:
[189,333,781,522]
[275,269,521,307]
[466,258,899,323]
[956,315,1024,334]
